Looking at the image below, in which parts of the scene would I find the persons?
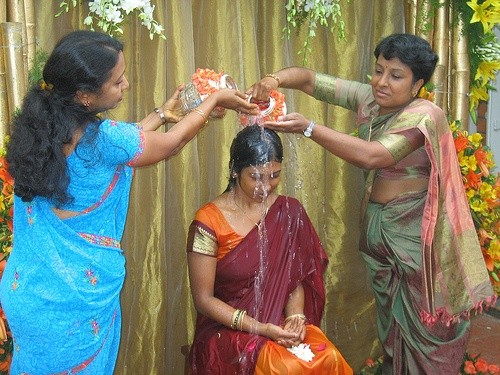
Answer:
[185,124,356,375]
[244,35,473,375]
[0,30,260,375]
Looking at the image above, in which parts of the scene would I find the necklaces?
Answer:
[231,188,269,236]
[368,115,385,142]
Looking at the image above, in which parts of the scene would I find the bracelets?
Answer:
[283,313,305,323]
[153,108,166,125]
[188,109,209,130]
[231,310,247,332]
[264,74,280,87]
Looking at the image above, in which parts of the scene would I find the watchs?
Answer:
[303,121,315,138]
